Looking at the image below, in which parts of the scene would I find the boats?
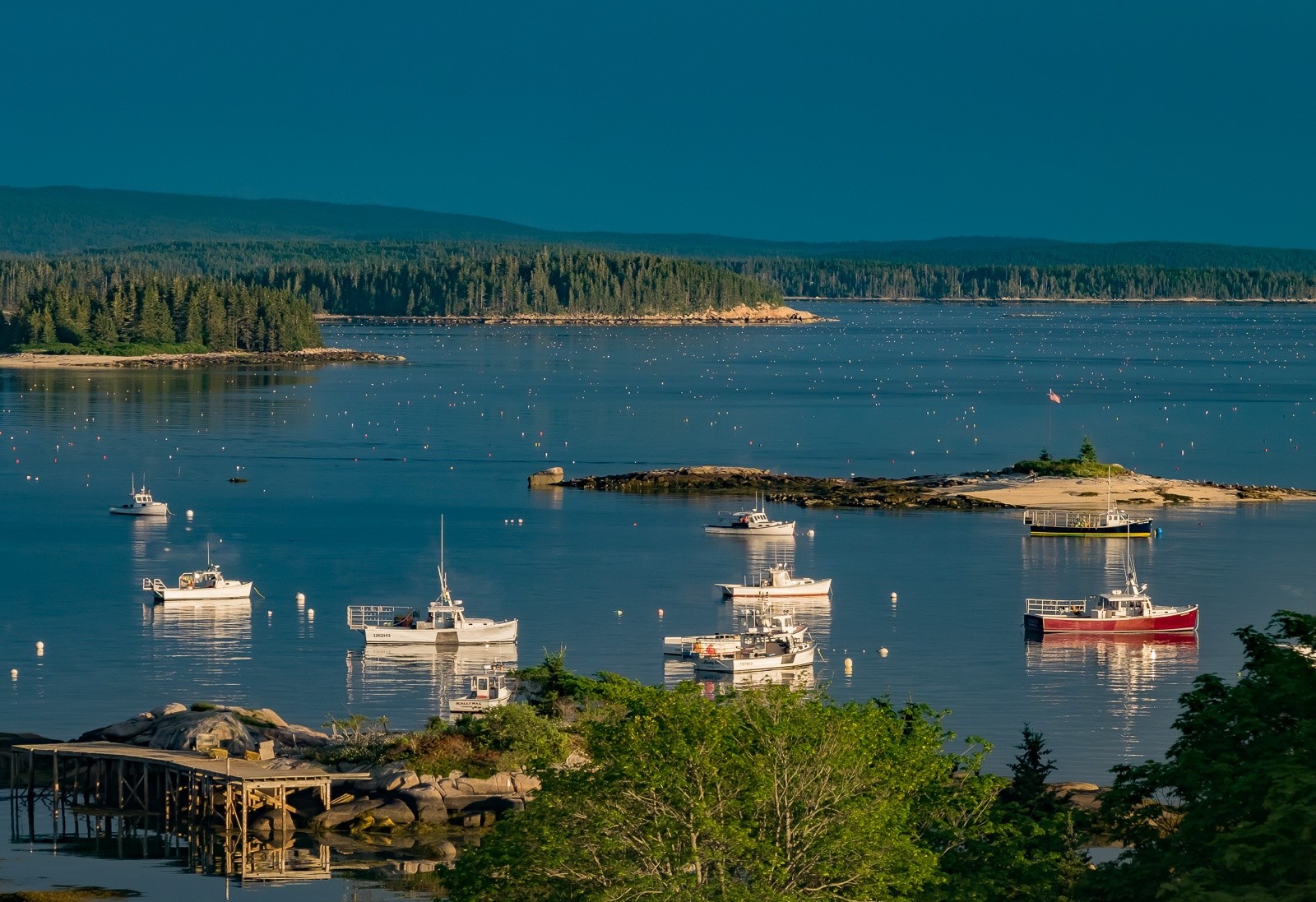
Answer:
[448,656,512,714]
[107,472,170,517]
[701,491,798,537]
[1023,515,1201,633]
[347,512,520,645]
[143,540,254,600]
[715,544,833,598]
[659,598,817,673]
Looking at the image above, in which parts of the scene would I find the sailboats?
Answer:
[1022,465,1162,540]
